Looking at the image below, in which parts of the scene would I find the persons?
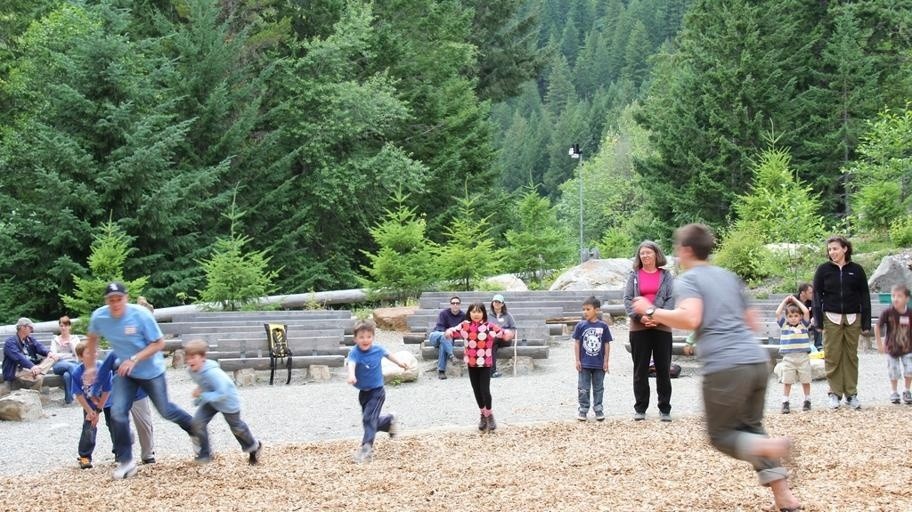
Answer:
[874,284,912,405]
[486,292,518,377]
[630,224,802,512]
[72,284,264,482]
[2,317,60,391]
[621,238,678,423]
[811,236,872,409]
[445,301,514,431]
[49,317,81,407]
[569,296,616,422]
[682,330,698,356]
[429,295,472,379]
[796,283,826,359]
[345,317,415,461]
[776,294,813,414]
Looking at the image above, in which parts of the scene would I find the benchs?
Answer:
[0,329,110,394]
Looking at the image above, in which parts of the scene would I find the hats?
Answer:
[16,317,34,328]
[103,282,126,297]
[492,294,504,303]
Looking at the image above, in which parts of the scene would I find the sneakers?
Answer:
[847,393,861,408]
[78,455,91,469]
[828,394,840,410]
[112,457,155,478]
[577,411,586,420]
[437,369,446,379]
[889,390,912,403]
[633,411,645,420]
[782,401,790,414]
[248,440,262,465]
[595,410,604,420]
[802,400,811,410]
[659,411,671,422]
[478,413,495,431]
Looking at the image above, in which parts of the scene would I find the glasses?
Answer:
[450,302,460,304]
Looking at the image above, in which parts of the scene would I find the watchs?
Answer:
[645,302,658,319]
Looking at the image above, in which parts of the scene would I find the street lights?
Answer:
[567,138,584,258]
